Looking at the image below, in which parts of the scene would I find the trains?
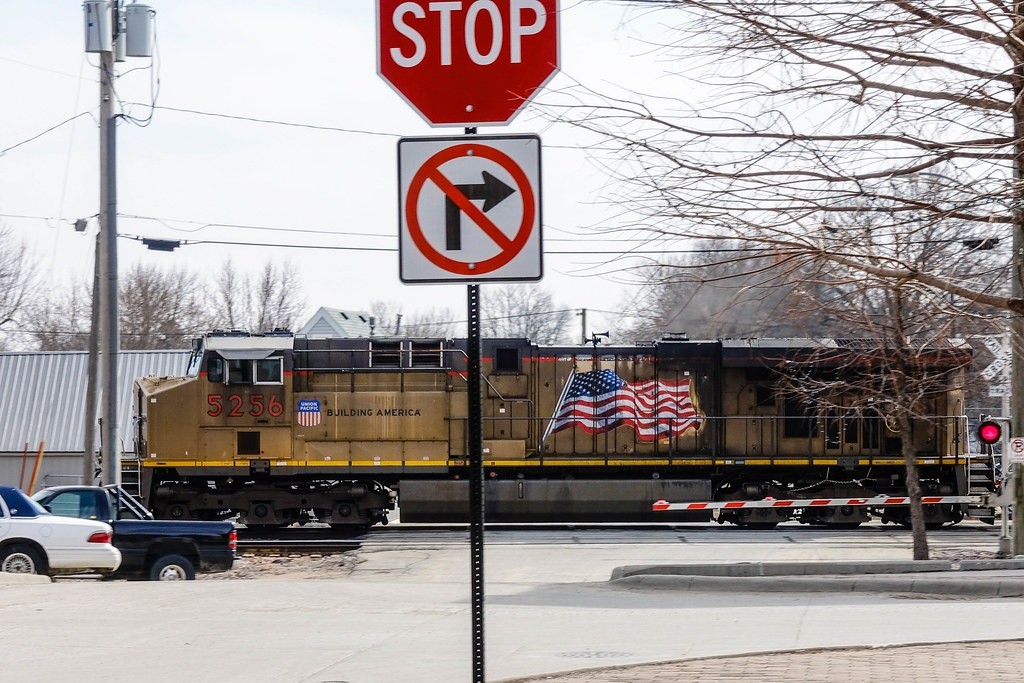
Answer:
[122,324,998,534]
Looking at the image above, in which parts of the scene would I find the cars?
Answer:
[0,487,124,583]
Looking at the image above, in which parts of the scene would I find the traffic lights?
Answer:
[975,421,1002,445]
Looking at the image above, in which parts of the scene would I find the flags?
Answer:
[549,369,704,441]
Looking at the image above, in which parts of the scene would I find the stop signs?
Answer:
[375,0,560,133]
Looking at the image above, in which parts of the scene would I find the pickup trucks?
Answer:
[23,484,240,583]
